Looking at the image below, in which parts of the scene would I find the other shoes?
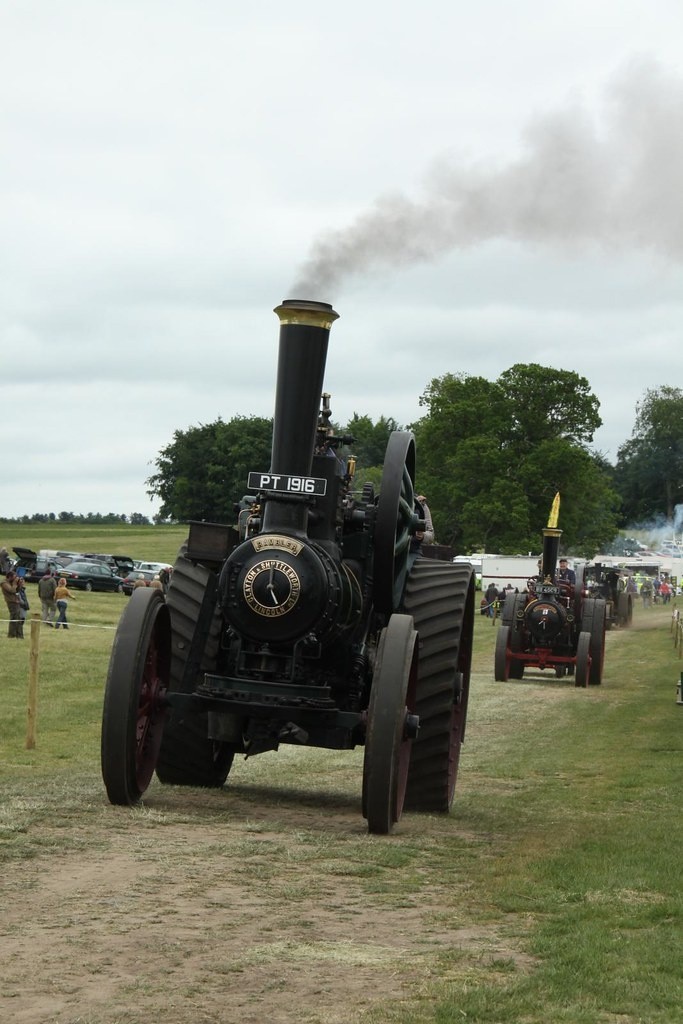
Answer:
[46,621,54,627]
[64,625,69,629]
[55,626,58,628]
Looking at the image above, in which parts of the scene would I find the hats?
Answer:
[560,559,566,562]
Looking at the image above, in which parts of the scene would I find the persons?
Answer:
[54,578,77,630]
[410,493,435,554]
[38,569,57,627]
[149,575,163,593]
[160,567,173,594]
[586,575,616,620]
[325,429,348,475]
[133,573,147,590]
[0,571,30,639]
[557,559,575,584]
[641,577,674,608]
[482,584,528,618]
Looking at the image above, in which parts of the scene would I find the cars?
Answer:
[112,555,173,596]
[10,546,65,582]
[52,562,124,592]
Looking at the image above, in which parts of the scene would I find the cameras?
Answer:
[17,578,20,582]
